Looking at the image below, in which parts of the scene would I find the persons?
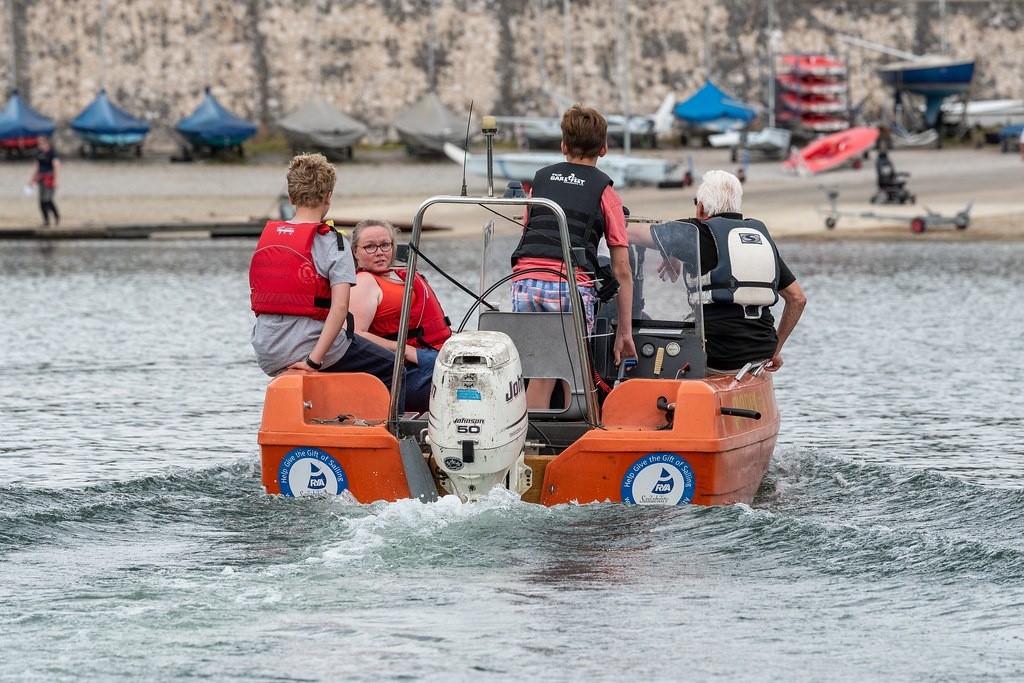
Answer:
[510,103,638,409]
[625,170,807,378]
[28,134,60,225]
[249,152,457,415]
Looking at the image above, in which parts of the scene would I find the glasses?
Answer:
[693,198,697,205]
[356,242,393,254]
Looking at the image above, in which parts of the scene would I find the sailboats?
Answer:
[836,31,975,99]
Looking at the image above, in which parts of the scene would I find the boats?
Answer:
[171,85,264,153]
[774,53,851,133]
[0,88,55,149]
[602,154,694,187]
[940,95,1023,138]
[67,87,152,147]
[669,79,754,133]
[784,125,879,173]
[390,89,481,160]
[256,101,779,507]
[710,125,790,155]
[486,94,682,149]
[441,141,628,187]
[276,100,367,150]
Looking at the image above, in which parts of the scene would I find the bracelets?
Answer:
[305,356,323,370]
